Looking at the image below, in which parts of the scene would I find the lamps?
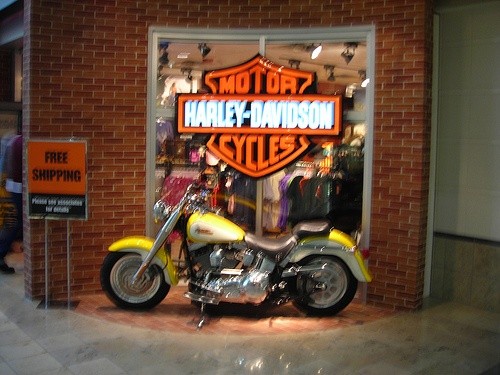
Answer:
[341,42,358,64]
[306,43,323,60]
[358,70,370,88]
[324,65,335,81]
[159,40,169,65]
[198,43,211,58]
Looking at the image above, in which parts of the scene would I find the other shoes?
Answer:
[0,260,15,274]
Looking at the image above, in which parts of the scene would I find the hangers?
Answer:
[281,146,363,184]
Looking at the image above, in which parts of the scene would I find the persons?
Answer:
[0,113,23,275]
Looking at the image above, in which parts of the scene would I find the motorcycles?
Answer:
[98,164,373,329]
